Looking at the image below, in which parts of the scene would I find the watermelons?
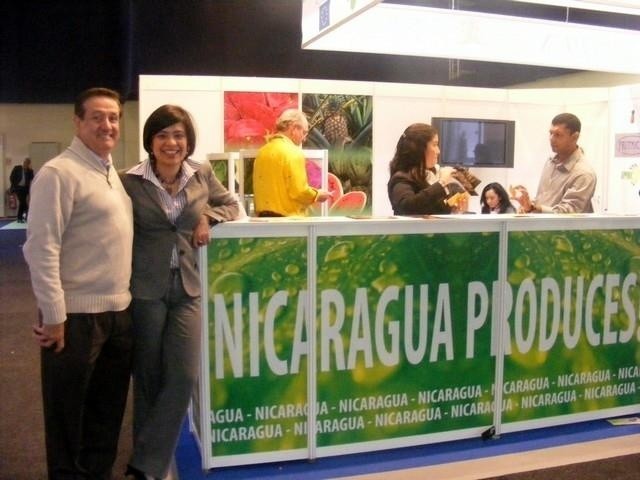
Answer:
[311,172,344,212]
[329,190,367,217]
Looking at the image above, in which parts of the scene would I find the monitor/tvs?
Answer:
[432,117,516,169]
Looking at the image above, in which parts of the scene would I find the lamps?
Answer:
[300,0,640,75]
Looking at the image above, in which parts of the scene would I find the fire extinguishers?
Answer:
[4,188,17,210]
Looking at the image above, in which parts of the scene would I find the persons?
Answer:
[20,87,136,480]
[113,104,240,479]
[387,123,453,214]
[512,113,597,214]
[480,182,516,214]
[251,108,336,218]
[9,158,34,223]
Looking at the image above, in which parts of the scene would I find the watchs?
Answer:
[525,203,536,213]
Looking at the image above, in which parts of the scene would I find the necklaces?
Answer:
[153,166,181,195]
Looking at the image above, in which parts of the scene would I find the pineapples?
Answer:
[321,95,348,146]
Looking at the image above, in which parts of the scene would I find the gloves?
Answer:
[442,177,466,194]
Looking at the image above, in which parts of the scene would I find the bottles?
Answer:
[236,193,255,216]
